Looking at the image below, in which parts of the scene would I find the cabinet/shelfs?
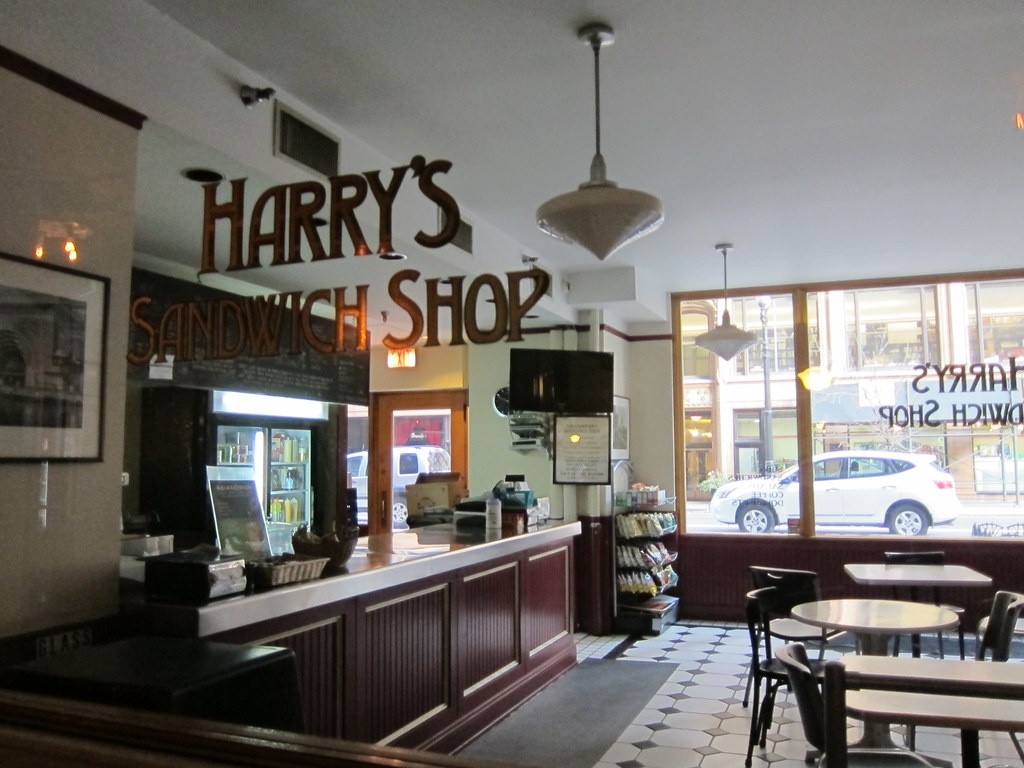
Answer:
[217,461,311,527]
[32,634,303,731]
[614,497,680,614]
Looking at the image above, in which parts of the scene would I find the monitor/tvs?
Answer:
[510,347,614,413]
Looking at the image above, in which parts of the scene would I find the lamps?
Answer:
[535,19,667,260]
[798,290,834,392]
[695,242,760,362]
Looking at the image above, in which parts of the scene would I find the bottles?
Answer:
[262,432,308,525]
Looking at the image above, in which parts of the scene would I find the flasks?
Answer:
[486,498,503,530]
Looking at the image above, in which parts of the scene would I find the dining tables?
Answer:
[790,598,960,767]
[842,563,993,659]
[815,652,1023,697]
[844,688,1023,733]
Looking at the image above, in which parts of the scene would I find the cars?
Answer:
[709,448,963,537]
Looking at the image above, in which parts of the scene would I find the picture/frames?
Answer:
[1,251,112,466]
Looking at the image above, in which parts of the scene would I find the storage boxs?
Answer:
[291,527,359,568]
[246,554,331,587]
[145,550,247,600]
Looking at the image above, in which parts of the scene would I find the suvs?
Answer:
[347,444,451,529]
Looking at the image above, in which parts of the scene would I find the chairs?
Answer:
[748,564,848,662]
[884,551,966,661]
[816,660,935,768]
[744,586,827,767]
[978,589,1024,763]
